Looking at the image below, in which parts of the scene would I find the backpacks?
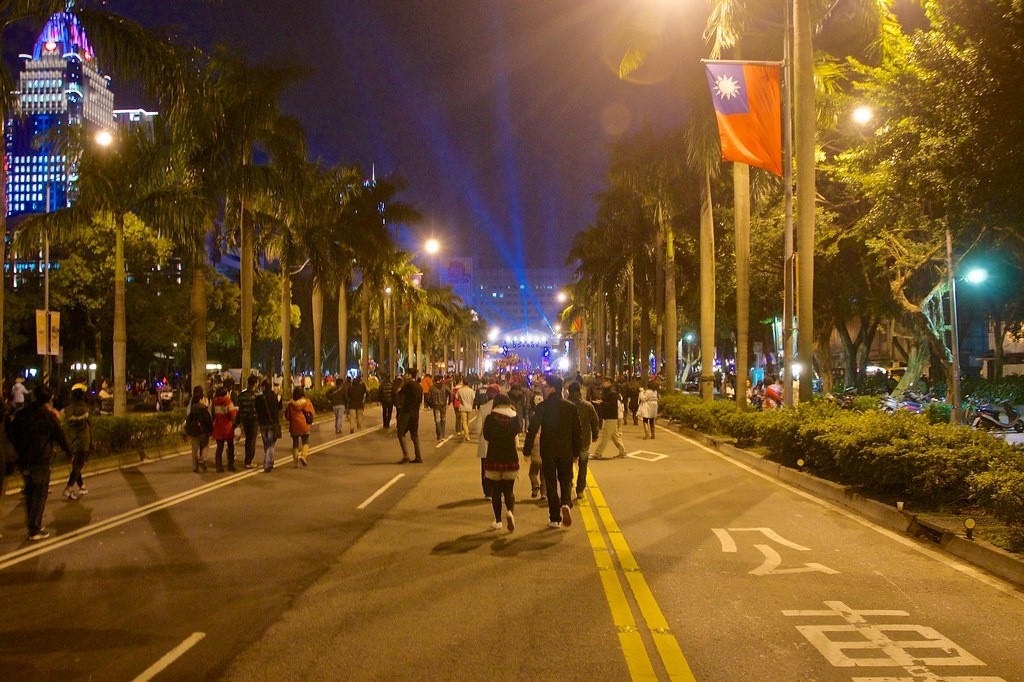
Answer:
[186,409,204,437]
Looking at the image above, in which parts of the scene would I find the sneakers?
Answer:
[79,486,89,494]
[62,488,77,499]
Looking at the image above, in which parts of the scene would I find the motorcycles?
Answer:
[826,387,857,412]
[917,386,945,403]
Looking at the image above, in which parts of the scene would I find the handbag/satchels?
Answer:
[636,405,643,418]
[274,424,282,439]
[306,412,313,424]
[234,425,242,442]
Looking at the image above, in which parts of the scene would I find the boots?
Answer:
[300,443,309,466]
[292,448,299,468]
[227,454,237,472]
[215,456,225,473]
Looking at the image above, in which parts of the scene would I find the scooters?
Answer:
[965,394,994,420]
[879,382,923,415]
[971,392,1024,433]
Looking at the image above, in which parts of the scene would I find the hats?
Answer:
[486,383,500,399]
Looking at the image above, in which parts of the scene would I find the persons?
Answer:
[482,393,521,531]
[715,369,722,391]
[523,375,584,528]
[0,366,659,499]
[745,376,786,412]
[7,384,75,540]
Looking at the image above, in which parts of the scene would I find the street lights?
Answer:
[45,130,112,402]
[946,228,1021,423]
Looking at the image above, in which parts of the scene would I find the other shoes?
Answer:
[398,458,410,464]
[613,454,628,459]
[549,518,561,527]
[492,521,502,529]
[643,433,655,440]
[484,495,492,501]
[409,456,423,463]
[577,491,585,499]
[541,494,546,499]
[28,525,49,539]
[506,510,515,531]
[562,504,571,526]
[438,438,444,443]
[588,453,603,459]
[245,463,258,468]
[192,460,207,472]
[532,487,540,497]
[456,430,464,436]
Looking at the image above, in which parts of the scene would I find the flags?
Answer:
[705,60,783,178]
